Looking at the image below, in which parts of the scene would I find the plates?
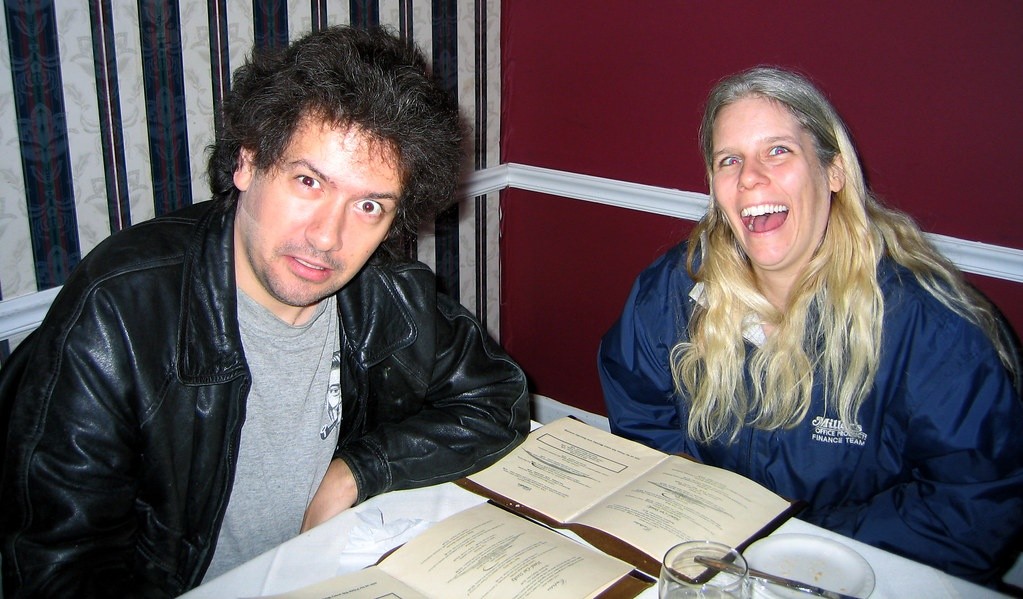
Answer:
[744,533,875,599]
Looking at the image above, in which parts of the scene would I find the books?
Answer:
[467,414,788,579]
[270,499,636,599]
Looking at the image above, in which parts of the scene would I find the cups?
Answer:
[659,541,748,599]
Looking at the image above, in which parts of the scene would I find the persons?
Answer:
[598,66,1022,584]
[1,25,532,599]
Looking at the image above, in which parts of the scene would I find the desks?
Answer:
[154,418,1013,599]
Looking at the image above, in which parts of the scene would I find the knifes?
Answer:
[693,554,863,599]
